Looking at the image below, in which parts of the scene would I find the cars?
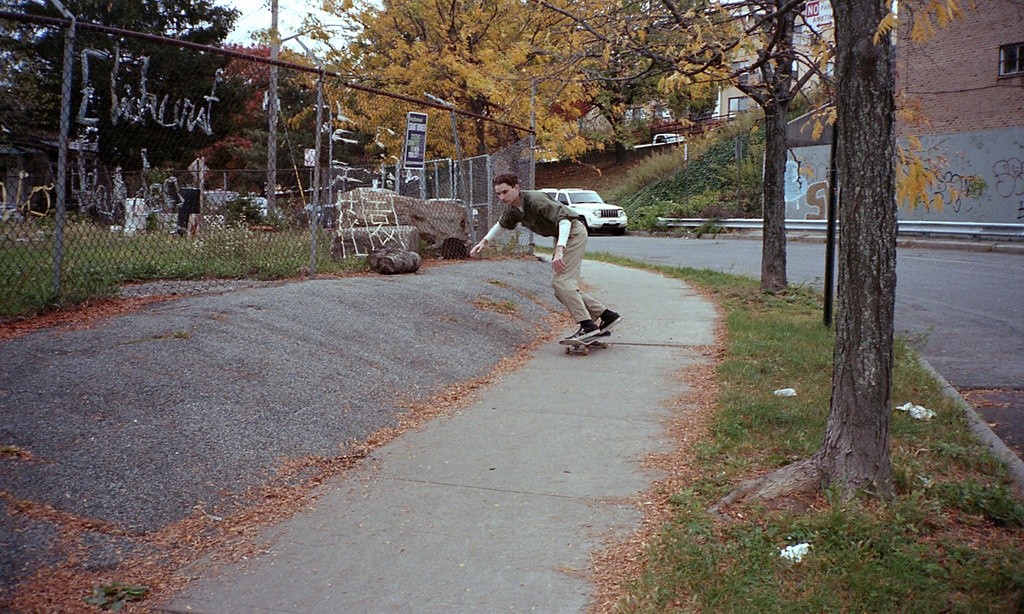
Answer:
[427,197,478,229]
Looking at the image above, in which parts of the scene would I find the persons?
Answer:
[469,173,622,339]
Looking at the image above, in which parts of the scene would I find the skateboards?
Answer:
[558,330,611,355]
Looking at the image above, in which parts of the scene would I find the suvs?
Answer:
[539,188,628,236]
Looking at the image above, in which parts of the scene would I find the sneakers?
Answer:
[598,312,622,336]
[564,323,600,341]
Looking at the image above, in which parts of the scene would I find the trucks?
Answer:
[634,134,684,149]
